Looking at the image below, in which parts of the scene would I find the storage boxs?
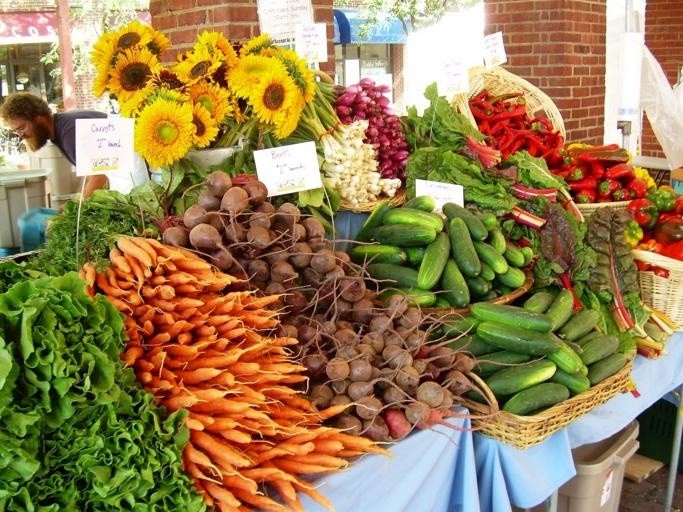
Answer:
[23,141,73,207]
[557,419,640,512]
[0,168,54,248]
[17,208,56,252]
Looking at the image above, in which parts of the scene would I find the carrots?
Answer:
[77,236,394,508]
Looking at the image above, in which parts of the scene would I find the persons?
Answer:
[0,91,108,200]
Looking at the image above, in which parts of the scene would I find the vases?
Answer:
[184,146,241,172]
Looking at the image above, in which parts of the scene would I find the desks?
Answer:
[274,406,479,512]
[473,331,683,512]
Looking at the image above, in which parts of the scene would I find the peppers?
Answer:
[468,87,683,265]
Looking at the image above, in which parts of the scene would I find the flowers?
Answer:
[89,19,317,173]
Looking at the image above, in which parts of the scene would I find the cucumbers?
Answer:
[350,194,627,414]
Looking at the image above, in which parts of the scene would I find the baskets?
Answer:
[575,200,633,220]
[372,240,535,321]
[452,359,633,449]
[336,185,409,214]
[629,248,683,332]
[448,65,566,146]
